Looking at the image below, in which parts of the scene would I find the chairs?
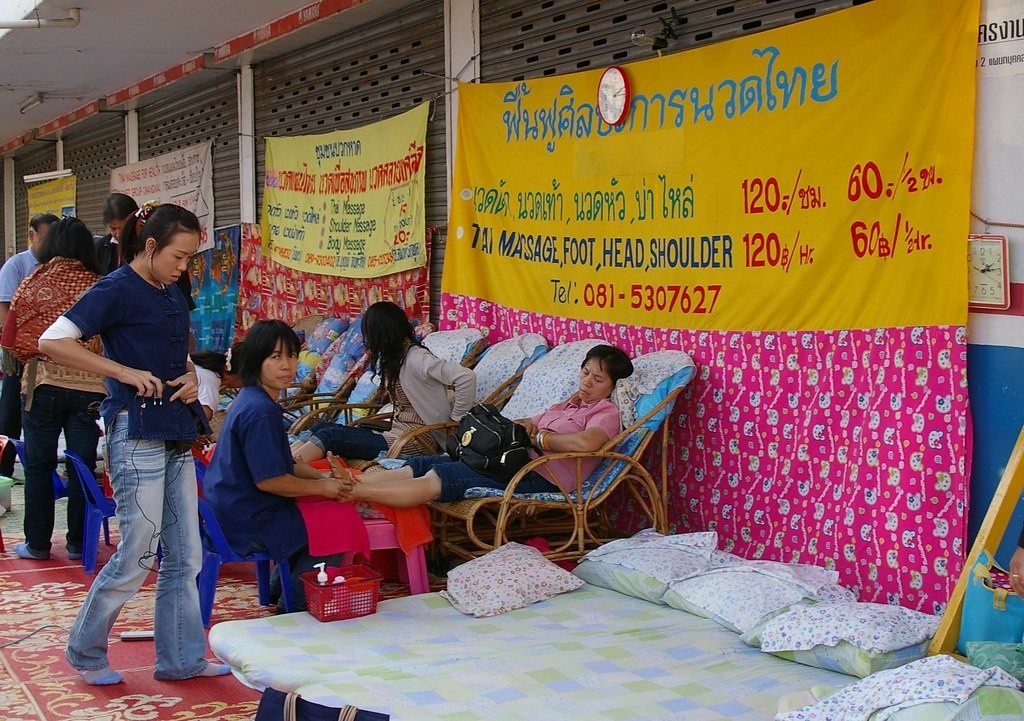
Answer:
[0,311,696,629]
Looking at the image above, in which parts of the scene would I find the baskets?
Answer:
[299,562,383,622]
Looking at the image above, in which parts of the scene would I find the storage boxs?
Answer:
[298,562,384,622]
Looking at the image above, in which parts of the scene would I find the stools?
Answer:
[337,518,431,595]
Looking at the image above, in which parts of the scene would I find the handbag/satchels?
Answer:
[445,402,543,484]
[358,418,392,432]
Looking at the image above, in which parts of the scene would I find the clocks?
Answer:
[966,233,1011,310]
[598,65,632,127]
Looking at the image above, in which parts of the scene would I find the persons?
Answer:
[0,193,141,560]
[288,345,634,509]
[1009,521,1024,598]
[185,342,244,421]
[291,300,476,461]
[202,319,361,612]
[33,201,232,684]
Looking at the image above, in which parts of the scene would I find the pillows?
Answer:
[739,597,944,680]
[659,559,858,634]
[870,652,1024,721]
[569,527,749,606]
[440,540,586,619]
[965,640,1024,691]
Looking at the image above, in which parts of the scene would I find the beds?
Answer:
[209,528,1024,721]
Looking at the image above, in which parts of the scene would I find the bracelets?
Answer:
[535,429,555,450]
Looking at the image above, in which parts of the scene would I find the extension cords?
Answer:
[119,631,155,641]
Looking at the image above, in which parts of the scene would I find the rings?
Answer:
[1012,575,1019,577]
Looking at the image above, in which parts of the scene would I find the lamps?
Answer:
[20,96,44,114]
